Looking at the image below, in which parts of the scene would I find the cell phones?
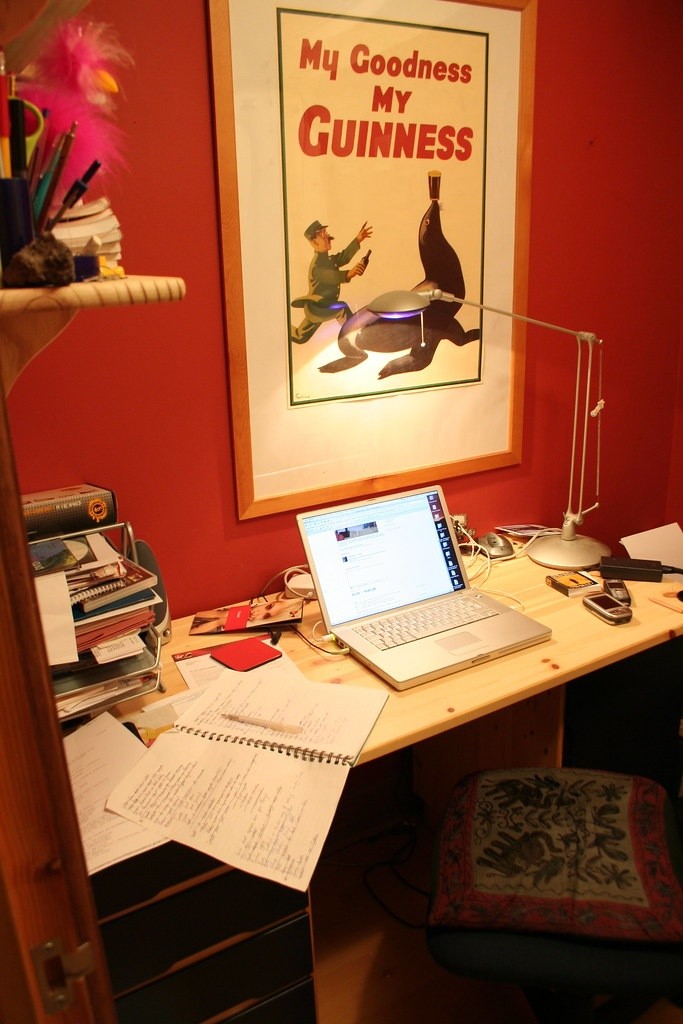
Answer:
[603,579,632,606]
[582,593,632,625]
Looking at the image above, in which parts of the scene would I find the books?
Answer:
[20,481,389,892]
[47,197,122,269]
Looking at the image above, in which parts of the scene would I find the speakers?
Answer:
[127,539,172,648]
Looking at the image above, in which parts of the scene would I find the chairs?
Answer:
[426,766,683,1024]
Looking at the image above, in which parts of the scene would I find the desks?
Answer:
[90,557,683,1024]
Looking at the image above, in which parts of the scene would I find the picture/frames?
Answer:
[206,0,538,523]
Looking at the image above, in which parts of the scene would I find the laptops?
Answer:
[295,485,552,691]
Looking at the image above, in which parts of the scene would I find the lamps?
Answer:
[366,288,610,571]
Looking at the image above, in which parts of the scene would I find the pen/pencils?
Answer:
[221,713,303,734]
[0,44,100,240]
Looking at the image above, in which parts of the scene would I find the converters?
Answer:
[599,555,663,582]
